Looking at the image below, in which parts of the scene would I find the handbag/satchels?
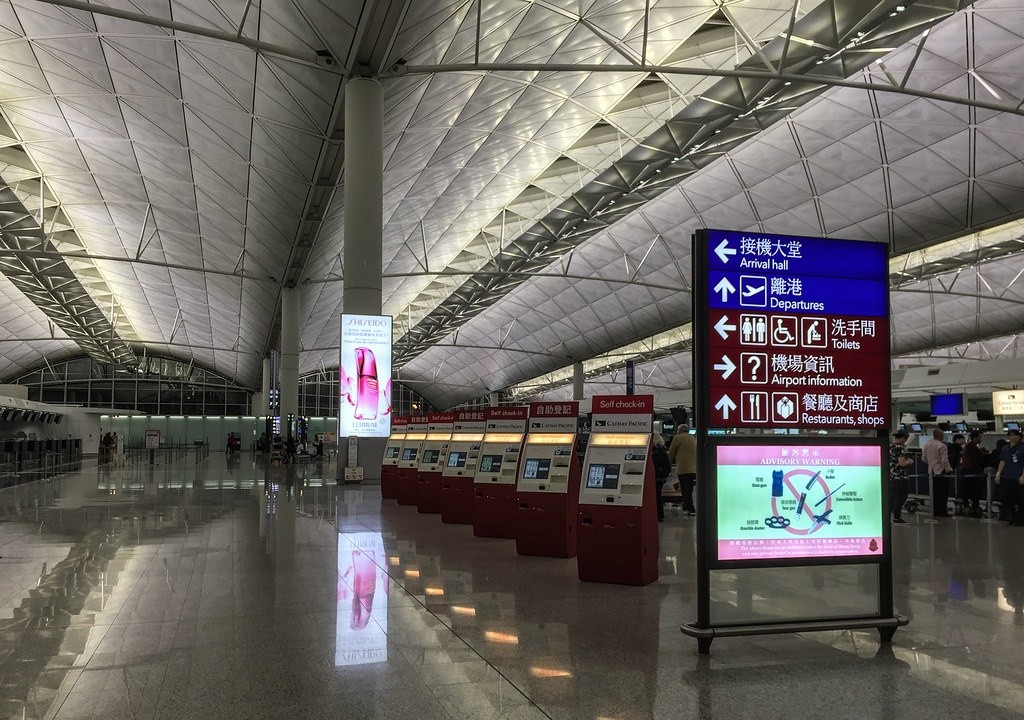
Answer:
[657,470,668,482]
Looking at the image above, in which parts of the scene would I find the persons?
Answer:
[652,434,671,522]
[889,430,914,525]
[100,427,118,461]
[922,428,1024,527]
[224,432,236,454]
[668,424,697,517]
[258,431,324,464]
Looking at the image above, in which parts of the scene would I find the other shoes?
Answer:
[893,518,905,523]
[684,510,688,514]
[690,511,696,515]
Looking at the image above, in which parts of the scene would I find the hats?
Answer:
[893,430,909,437]
[678,424,689,431]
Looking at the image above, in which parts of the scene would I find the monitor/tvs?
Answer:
[900,423,906,430]
[524,459,552,479]
[587,465,620,489]
[1006,422,1021,430]
[955,423,968,431]
[386,447,468,467]
[911,423,922,432]
[480,455,503,472]
